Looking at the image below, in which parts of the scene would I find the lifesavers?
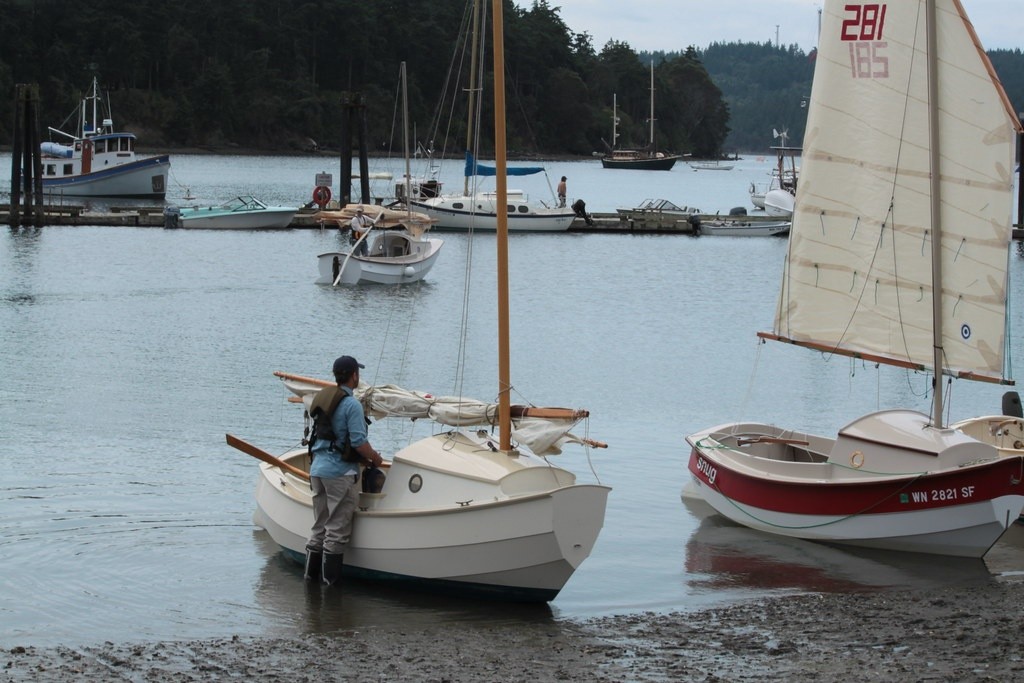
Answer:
[312,185,331,205]
[849,450,865,469]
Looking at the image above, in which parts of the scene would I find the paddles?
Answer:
[333,210,382,286]
[225,432,309,482]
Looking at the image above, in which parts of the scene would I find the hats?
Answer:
[333,356,365,376]
[356,205,364,212]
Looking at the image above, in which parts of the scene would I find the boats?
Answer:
[684,160,735,171]
[616,125,804,235]
[162,193,300,229]
[21,76,170,200]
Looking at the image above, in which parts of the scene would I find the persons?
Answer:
[350,205,376,257]
[558,176,567,208]
[302,356,383,590]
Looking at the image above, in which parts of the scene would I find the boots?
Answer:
[305,546,322,583]
[322,550,344,585]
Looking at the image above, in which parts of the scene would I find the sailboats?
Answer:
[593,57,692,171]
[350,0,578,232]
[688,0,1023,564]
[314,61,445,287]
[222,0,615,605]
